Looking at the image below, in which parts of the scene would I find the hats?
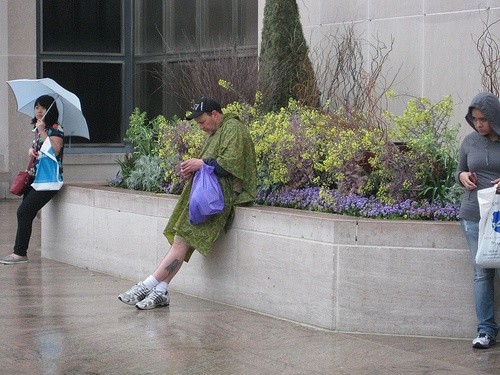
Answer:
[185,98,221,121]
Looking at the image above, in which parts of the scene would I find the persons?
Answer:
[117,99,255,309]
[0,95,64,264]
[455,92,500,349]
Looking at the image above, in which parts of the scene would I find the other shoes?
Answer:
[0,253,28,264]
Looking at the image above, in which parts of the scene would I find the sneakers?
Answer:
[135,287,170,310]
[118,283,152,305]
[471,333,497,348]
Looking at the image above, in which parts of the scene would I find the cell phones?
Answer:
[469,172,477,185]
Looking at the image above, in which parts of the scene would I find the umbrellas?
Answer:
[6,77,91,141]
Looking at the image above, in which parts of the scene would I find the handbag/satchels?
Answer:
[31,135,65,191]
[8,170,33,197]
[474,184,500,269]
[188,162,224,225]
